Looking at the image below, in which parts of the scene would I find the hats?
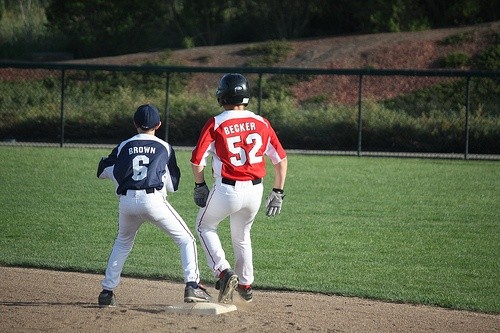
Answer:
[133,103,160,131]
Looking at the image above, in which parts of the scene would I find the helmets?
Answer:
[216,73,250,108]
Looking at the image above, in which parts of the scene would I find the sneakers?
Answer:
[98,291,118,307]
[215,268,239,304]
[184,284,213,303]
[235,284,253,303]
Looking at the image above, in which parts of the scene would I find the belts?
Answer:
[121,188,154,195]
[222,177,261,186]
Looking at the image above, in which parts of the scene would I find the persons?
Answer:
[96,103,214,308]
[189,73,288,306]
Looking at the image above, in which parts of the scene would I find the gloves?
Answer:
[194,181,210,208]
[265,188,286,217]
